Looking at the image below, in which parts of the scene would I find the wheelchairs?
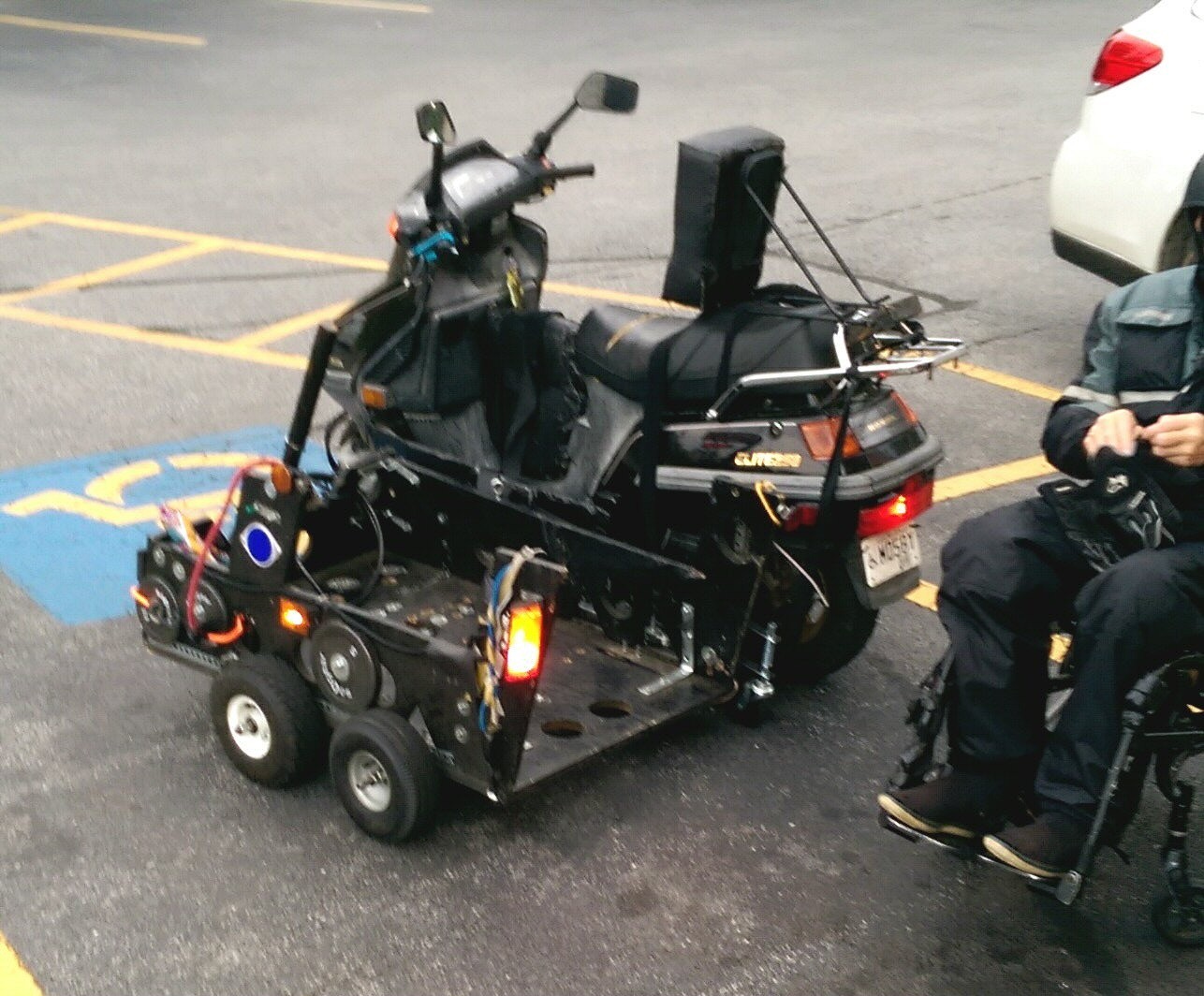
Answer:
[879,638,1204,957]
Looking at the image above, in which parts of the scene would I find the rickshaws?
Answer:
[132,71,974,845]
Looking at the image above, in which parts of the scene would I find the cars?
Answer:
[1047,1,1204,291]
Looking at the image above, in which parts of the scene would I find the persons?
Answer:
[877,196,1204,880]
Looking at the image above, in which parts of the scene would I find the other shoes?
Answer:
[981,813,1092,882]
[875,771,1018,837]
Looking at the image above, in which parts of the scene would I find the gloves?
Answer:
[1096,438,1182,550]
[1037,478,1122,573]
